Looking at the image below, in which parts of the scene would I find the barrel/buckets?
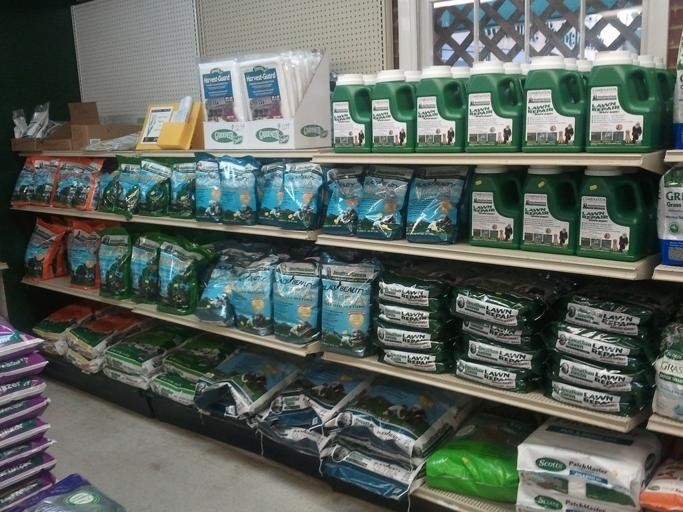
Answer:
[415,67,467,154]
[465,62,522,154]
[521,56,587,153]
[403,71,419,97]
[450,68,470,93]
[576,162,651,262]
[565,57,590,87]
[586,53,663,154]
[330,74,371,154]
[633,53,677,153]
[520,164,578,256]
[467,164,520,250]
[371,72,415,154]
[502,62,528,93]
[362,73,376,96]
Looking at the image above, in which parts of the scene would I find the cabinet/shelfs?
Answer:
[646,148,683,439]
[11,149,663,512]
